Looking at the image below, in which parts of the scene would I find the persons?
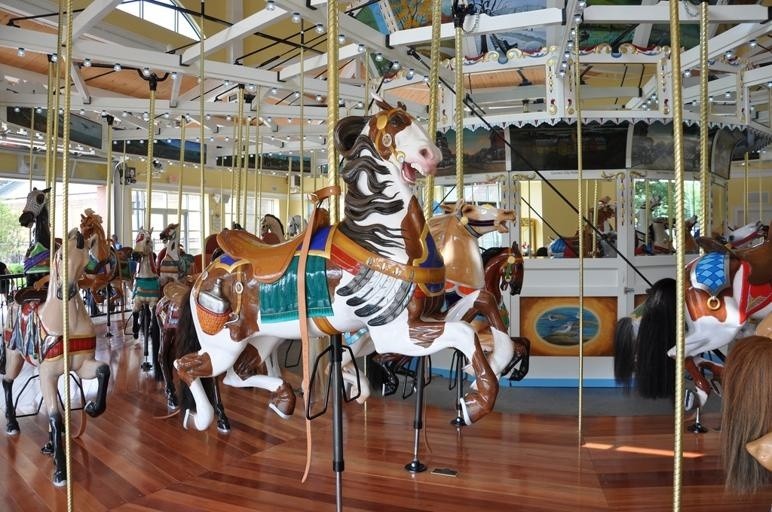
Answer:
[110,234,122,250]
[0,262,15,325]
